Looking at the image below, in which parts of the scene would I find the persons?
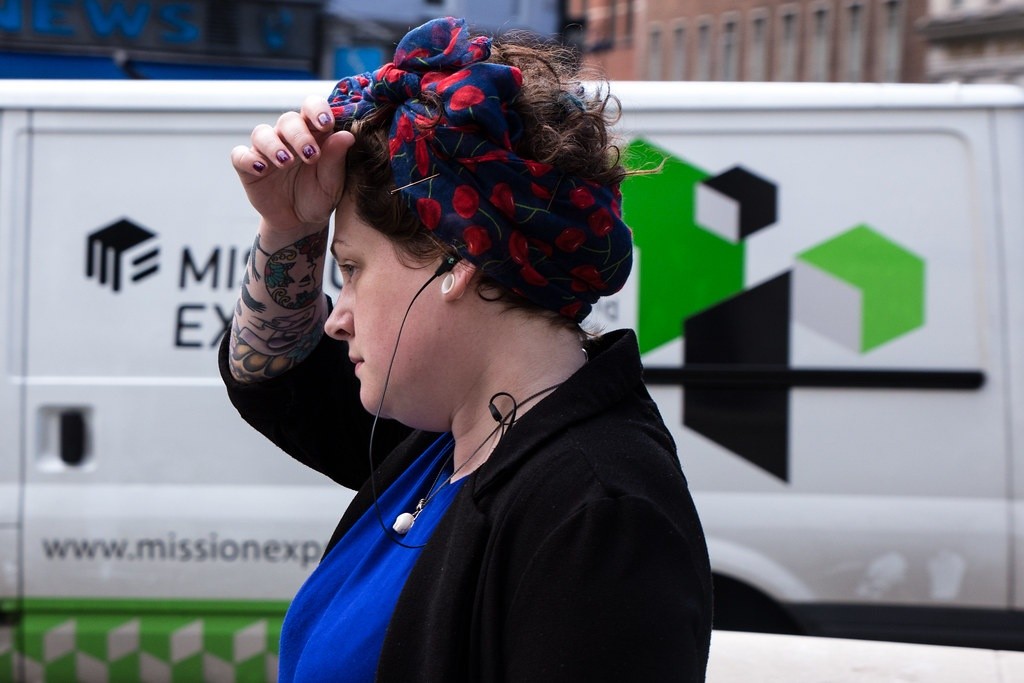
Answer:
[219,0,713,683]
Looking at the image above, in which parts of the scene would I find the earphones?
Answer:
[435,252,461,278]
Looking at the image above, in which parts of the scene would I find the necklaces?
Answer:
[392,347,589,532]
